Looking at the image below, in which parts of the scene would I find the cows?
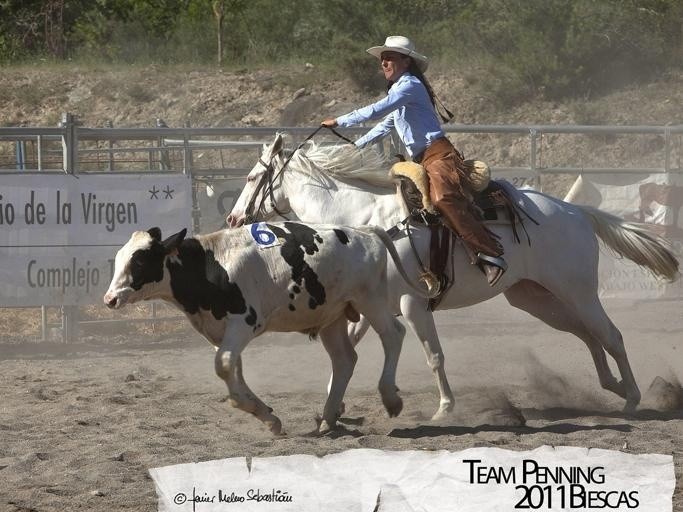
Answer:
[103,219,441,435]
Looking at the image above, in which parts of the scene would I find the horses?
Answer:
[224,133,682,423]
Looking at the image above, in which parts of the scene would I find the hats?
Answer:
[365,35,429,74]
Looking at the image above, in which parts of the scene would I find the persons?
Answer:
[322,35,505,286]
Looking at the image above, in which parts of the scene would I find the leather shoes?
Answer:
[481,256,505,287]
